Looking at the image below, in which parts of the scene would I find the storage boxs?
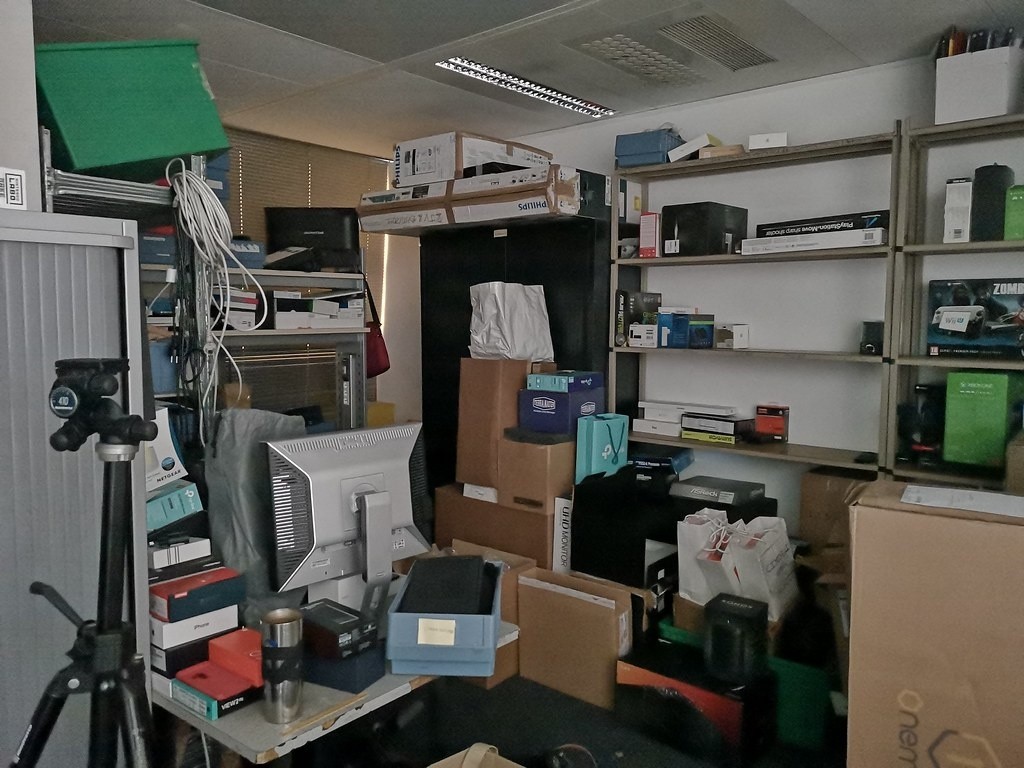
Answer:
[136,34,1024,768]
[34,38,231,182]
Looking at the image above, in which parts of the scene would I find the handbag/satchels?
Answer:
[575,413,629,485]
[677,507,800,622]
[343,268,390,382]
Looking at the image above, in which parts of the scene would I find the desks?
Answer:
[140,620,522,768]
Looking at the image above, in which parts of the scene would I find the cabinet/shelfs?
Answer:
[907,106,1024,489]
[617,132,897,478]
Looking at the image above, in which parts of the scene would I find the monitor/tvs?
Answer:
[259,422,436,640]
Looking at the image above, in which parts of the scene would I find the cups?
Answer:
[259,608,303,725]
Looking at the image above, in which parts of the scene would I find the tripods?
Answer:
[10,358,158,768]
